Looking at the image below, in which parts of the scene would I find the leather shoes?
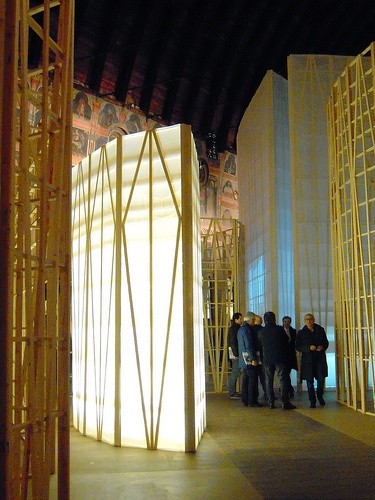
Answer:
[269,403,276,409]
[281,401,296,410]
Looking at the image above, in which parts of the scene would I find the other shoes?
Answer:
[317,395,325,406]
[289,392,294,399]
[244,401,264,407]
[229,392,242,400]
[310,399,317,408]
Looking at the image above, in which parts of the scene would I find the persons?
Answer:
[256,311,296,410]
[227,312,243,400]
[237,312,264,407]
[295,314,329,408]
[251,316,278,400]
[281,316,302,400]
